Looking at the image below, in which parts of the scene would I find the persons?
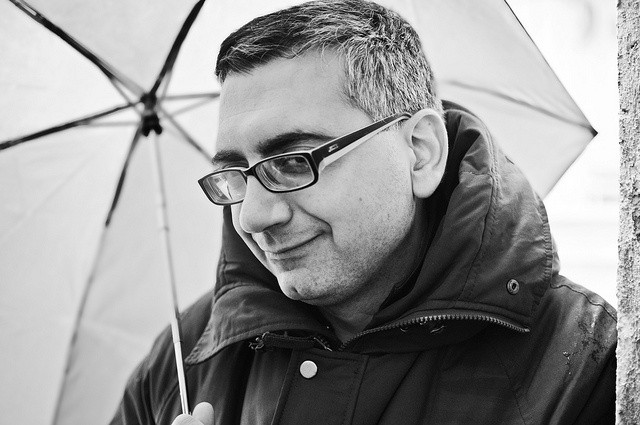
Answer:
[108,0,618,424]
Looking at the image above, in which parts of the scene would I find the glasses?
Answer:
[197,112,411,205]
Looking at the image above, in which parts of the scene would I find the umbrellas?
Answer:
[0,1,599,424]
